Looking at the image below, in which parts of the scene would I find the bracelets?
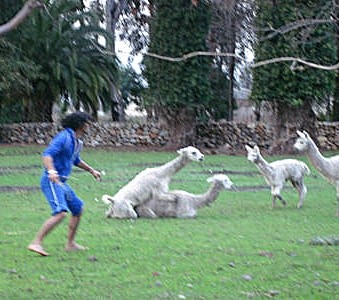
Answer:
[47,169,58,174]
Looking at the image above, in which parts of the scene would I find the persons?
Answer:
[28,112,101,256]
